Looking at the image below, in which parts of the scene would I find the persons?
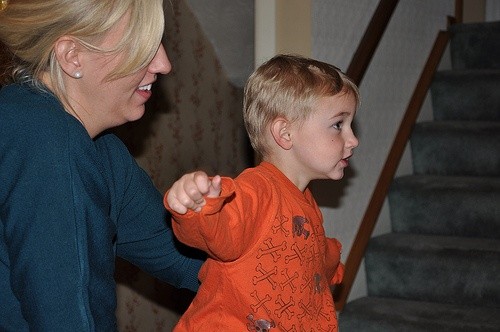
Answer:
[1,1,206,331]
[162,53,360,332]
